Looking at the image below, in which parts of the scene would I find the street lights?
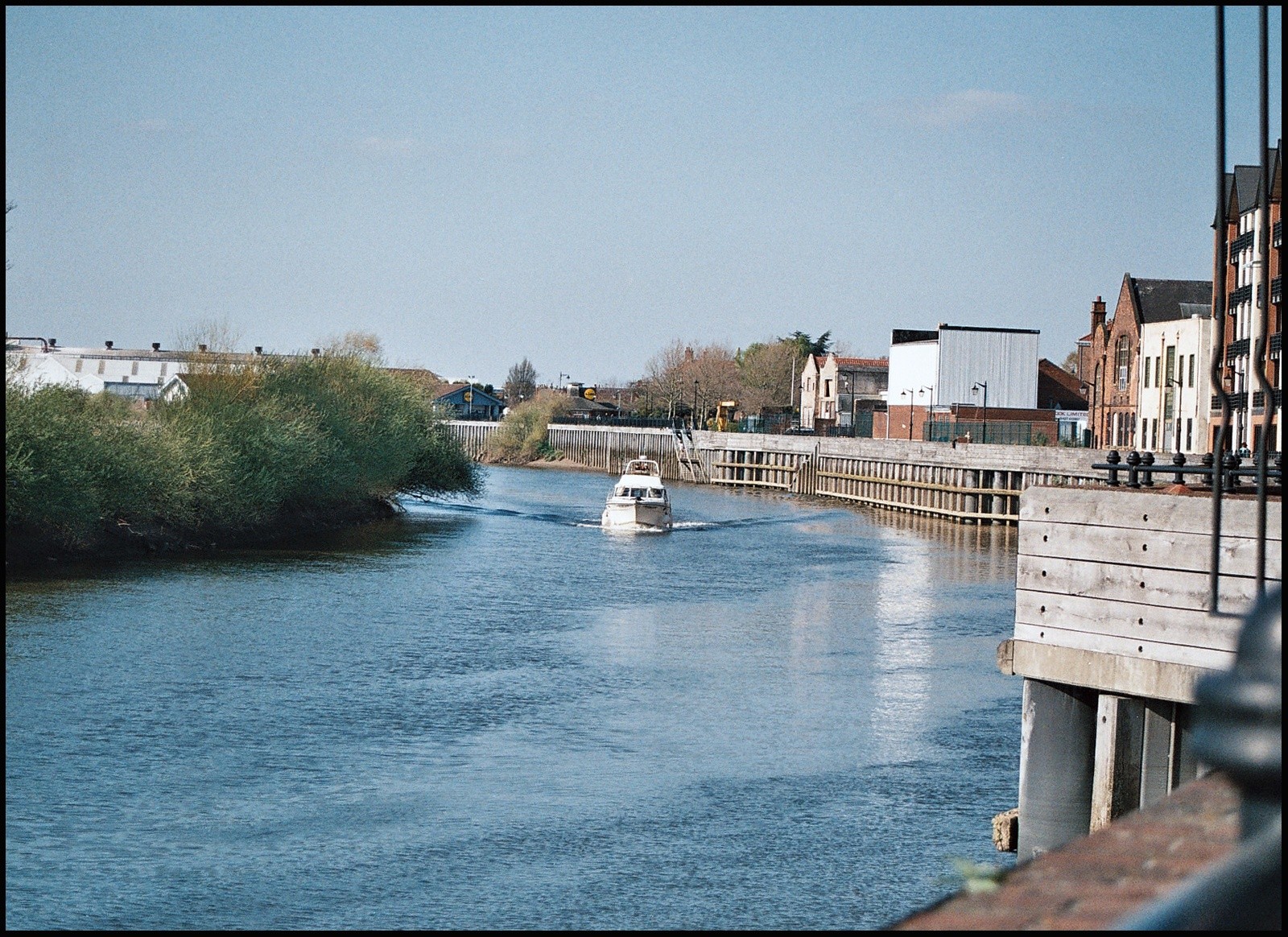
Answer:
[971,379,987,443]
[919,384,933,441]
[901,386,913,440]
[1224,367,1245,456]
[1164,376,1183,452]
[1079,361,1099,448]
[559,372,570,389]
[798,382,804,432]
[693,379,699,419]
[468,374,475,414]
[835,370,854,424]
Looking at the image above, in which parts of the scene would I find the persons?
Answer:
[634,463,641,470]
[1239,441,1251,458]
[641,464,650,476]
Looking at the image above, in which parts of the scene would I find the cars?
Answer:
[784,425,814,435]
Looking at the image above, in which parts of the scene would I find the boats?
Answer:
[601,454,673,532]
